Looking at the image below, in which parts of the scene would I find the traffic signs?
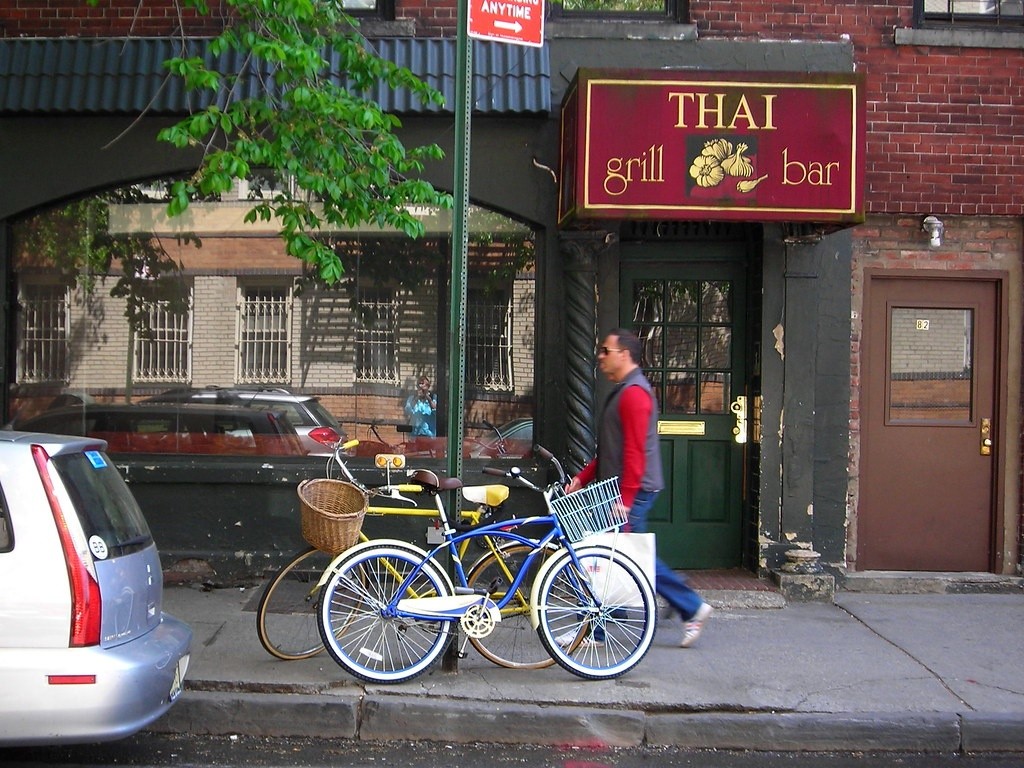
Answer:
[468,0,544,48]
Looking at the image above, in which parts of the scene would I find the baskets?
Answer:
[297,478,369,557]
[550,476,628,542]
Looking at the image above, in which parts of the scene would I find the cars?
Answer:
[14,384,356,458]
[0,432,192,748]
[402,418,533,460]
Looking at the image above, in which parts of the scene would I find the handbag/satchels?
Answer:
[579,517,656,607]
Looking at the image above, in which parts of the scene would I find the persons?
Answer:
[404,377,437,437]
[556,328,713,648]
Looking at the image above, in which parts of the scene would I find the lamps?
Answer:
[922,215,944,251]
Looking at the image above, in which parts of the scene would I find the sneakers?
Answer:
[554,627,605,648]
[682,602,714,647]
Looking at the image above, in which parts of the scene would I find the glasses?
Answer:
[600,347,622,356]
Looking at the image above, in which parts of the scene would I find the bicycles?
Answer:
[257,437,592,670]
[316,444,658,684]
[366,416,505,459]
